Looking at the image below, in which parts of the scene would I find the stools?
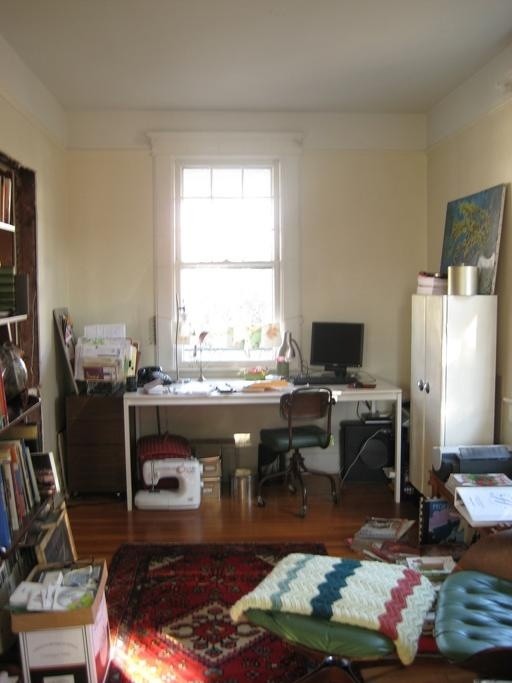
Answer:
[230,551,432,682]
[432,528,512,683]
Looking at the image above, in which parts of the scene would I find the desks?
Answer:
[425,469,505,538]
[123,373,401,512]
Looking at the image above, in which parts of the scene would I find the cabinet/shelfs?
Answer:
[64,339,140,499]
[407,293,498,501]
[0,151,52,564]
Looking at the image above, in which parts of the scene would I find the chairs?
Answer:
[254,386,341,517]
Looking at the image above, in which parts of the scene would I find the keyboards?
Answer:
[292,375,359,385]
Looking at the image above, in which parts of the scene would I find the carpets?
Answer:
[102,541,335,683]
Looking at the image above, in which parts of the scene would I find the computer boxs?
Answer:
[340,420,395,481]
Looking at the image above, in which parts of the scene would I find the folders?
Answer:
[453,485,512,529]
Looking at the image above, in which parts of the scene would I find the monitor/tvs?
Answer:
[310,321,365,378]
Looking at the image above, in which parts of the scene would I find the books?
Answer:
[416,275,447,294]
[444,473,512,495]
[74,335,138,382]
[356,517,416,542]
[9,565,101,610]
[0,422,60,553]
[0,173,12,224]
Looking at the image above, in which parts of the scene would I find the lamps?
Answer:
[277,331,304,385]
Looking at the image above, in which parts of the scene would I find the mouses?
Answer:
[355,381,364,388]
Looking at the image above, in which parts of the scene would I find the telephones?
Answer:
[137,365,173,387]
